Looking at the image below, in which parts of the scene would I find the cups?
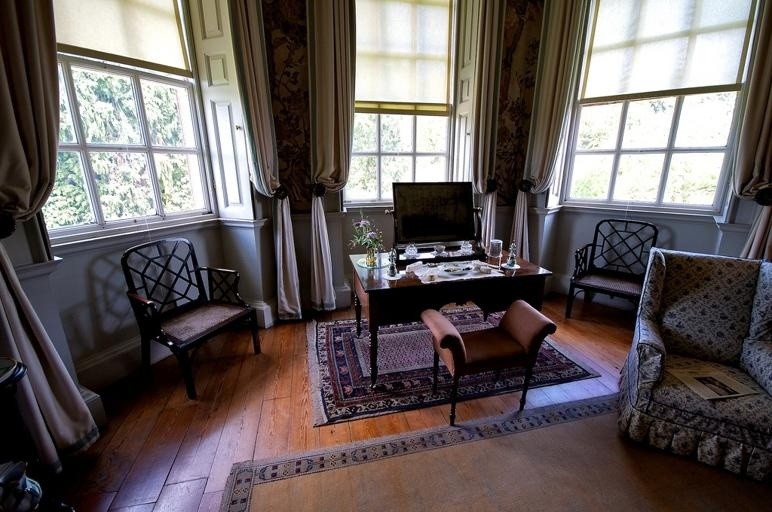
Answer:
[490,240,502,256]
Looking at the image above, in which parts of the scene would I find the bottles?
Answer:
[405,242,417,256]
[507,240,517,267]
[461,241,472,253]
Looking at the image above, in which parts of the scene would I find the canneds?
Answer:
[489,239,502,257]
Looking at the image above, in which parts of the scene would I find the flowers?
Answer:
[345,208,389,263]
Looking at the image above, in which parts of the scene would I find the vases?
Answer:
[366,246,380,268]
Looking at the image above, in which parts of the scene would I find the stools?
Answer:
[421,298,557,427]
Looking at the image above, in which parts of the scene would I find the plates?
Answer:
[443,263,474,276]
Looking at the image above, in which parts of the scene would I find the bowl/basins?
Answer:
[433,245,445,253]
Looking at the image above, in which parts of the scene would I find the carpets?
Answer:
[306,315,602,428]
[216,392,772,512]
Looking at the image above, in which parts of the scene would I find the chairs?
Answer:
[565,218,660,319]
[618,247,772,484]
[120,237,261,397]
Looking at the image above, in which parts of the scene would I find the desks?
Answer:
[346,243,555,389]
